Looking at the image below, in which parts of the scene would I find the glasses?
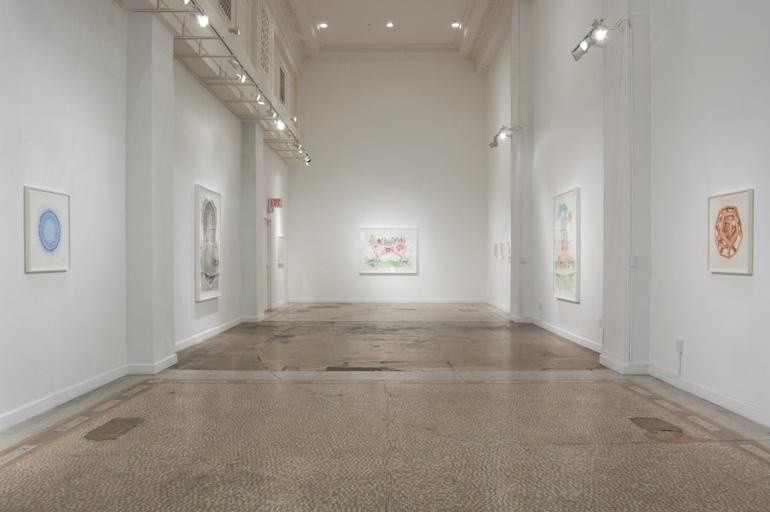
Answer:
[490,125,525,149]
[570,16,632,63]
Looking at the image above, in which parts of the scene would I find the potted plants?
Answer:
[23,185,71,275]
[552,188,583,306]
[355,225,421,277]
[193,184,223,304]
[706,190,757,278]
